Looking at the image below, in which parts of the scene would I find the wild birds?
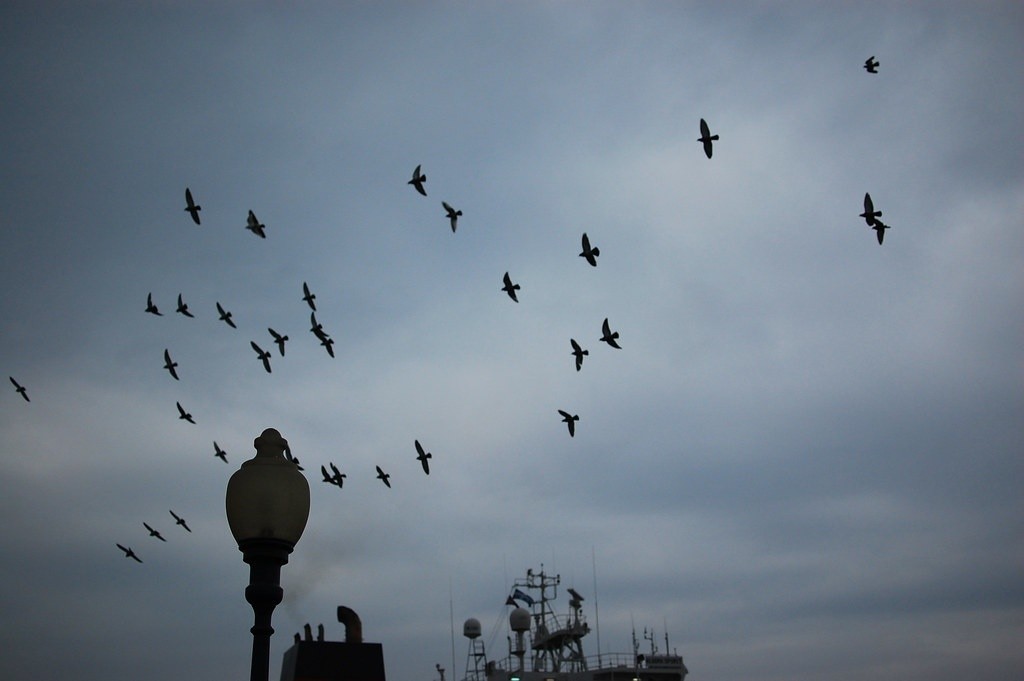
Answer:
[860,53,883,76]
[175,401,197,425]
[557,409,580,437]
[598,318,624,349]
[168,509,194,534]
[578,233,600,267]
[213,440,229,464]
[143,159,463,359]
[329,462,347,488]
[414,439,432,475]
[8,376,32,403]
[251,341,272,373]
[375,465,391,488]
[115,543,144,563]
[320,464,338,486]
[570,338,591,370]
[698,118,897,247]
[501,271,521,303]
[142,521,167,542]
[163,348,179,380]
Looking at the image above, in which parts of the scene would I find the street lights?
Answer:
[222,427,311,681]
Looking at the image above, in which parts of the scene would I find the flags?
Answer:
[506,597,519,608]
[513,589,533,607]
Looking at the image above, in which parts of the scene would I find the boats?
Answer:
[434,562,691,680]
